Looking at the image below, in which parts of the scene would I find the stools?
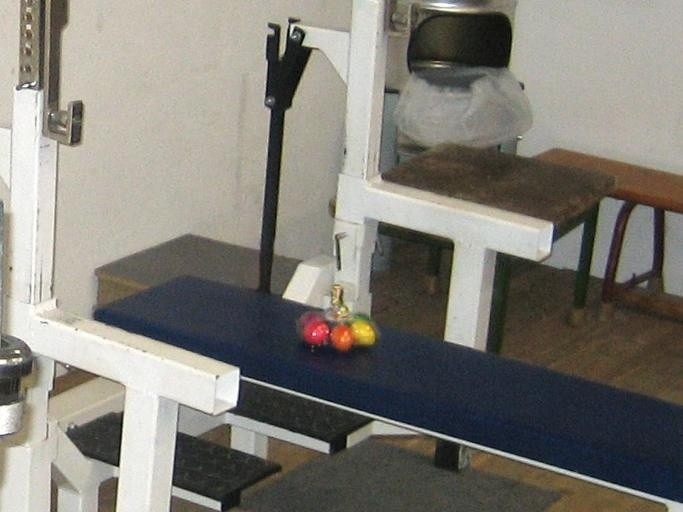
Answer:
[222,379,374,460]
[66,411,280,512]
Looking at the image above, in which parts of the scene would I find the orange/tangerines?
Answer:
[330,326,353,351]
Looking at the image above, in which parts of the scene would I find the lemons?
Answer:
[350,321,375,346]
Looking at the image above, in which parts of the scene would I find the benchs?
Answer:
[531,148,682,323]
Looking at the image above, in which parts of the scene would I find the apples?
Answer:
[304,319,329,344]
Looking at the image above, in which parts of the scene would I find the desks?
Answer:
[95,276,681,512]
[375,142,613,354]
